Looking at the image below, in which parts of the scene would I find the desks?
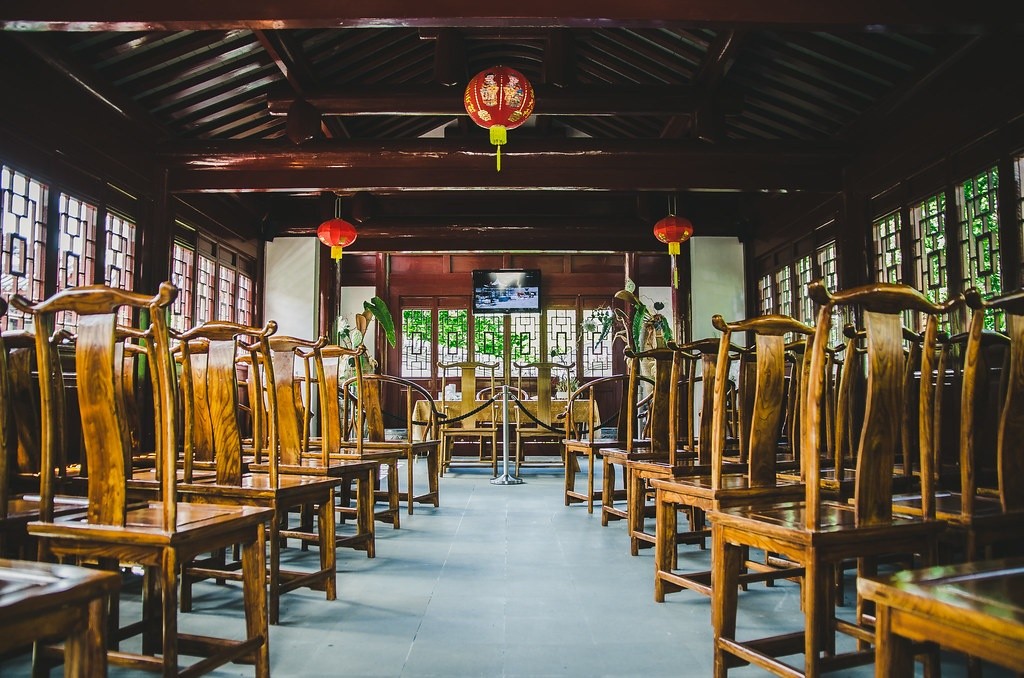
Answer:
[412,399,601,469]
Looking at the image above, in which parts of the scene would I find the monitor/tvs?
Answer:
[470,267,542,314]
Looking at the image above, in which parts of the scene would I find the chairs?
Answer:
[1,281,1024,678]
